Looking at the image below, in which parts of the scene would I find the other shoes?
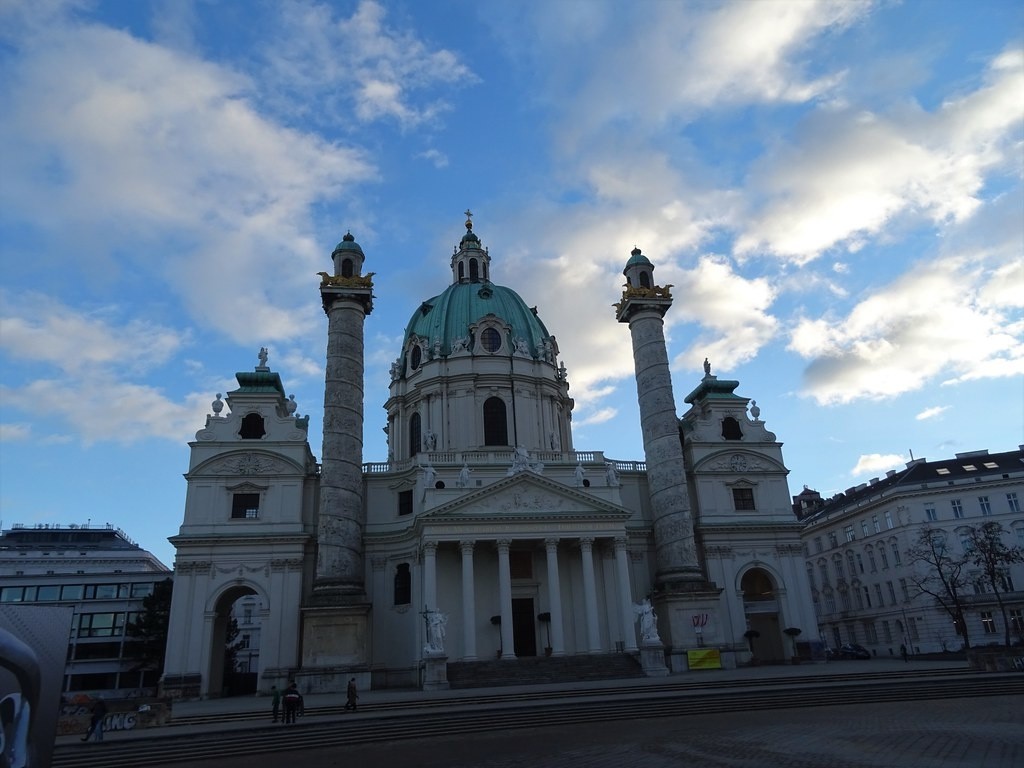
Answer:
[282,720,284,723]
[272,719,278,723]
[286,721,291,724]
[343,707,348,712]
[80,738,88,741]
[292,721,296,723]
[296,717,303,720]
[353,709,357,712]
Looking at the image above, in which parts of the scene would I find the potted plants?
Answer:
[783,628,802,665]
[490,615,503,659]
[537,612,553,657]
[743,630,761,667]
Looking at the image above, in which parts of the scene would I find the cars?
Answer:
[839,643,870,660]
[825,647,840,660]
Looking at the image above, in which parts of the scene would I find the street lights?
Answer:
[899,599,915,655]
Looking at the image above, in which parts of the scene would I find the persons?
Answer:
[900,644,908,663]
[513,445,528,468]
[281,683,304,724]
[271,685,280,723]
[80,695,105,742]
[551,432,559,448]
[454,336,467,352]
[423,429,434,451]
[512,336,528,353]
[258,348,268,366]
[429,608,445,649]
[607,462,619,484]
[419,463,438,487]
[640,599,659,640]
[574,463,585,484]
[345,678,359,710]
[459,464,471,487]
